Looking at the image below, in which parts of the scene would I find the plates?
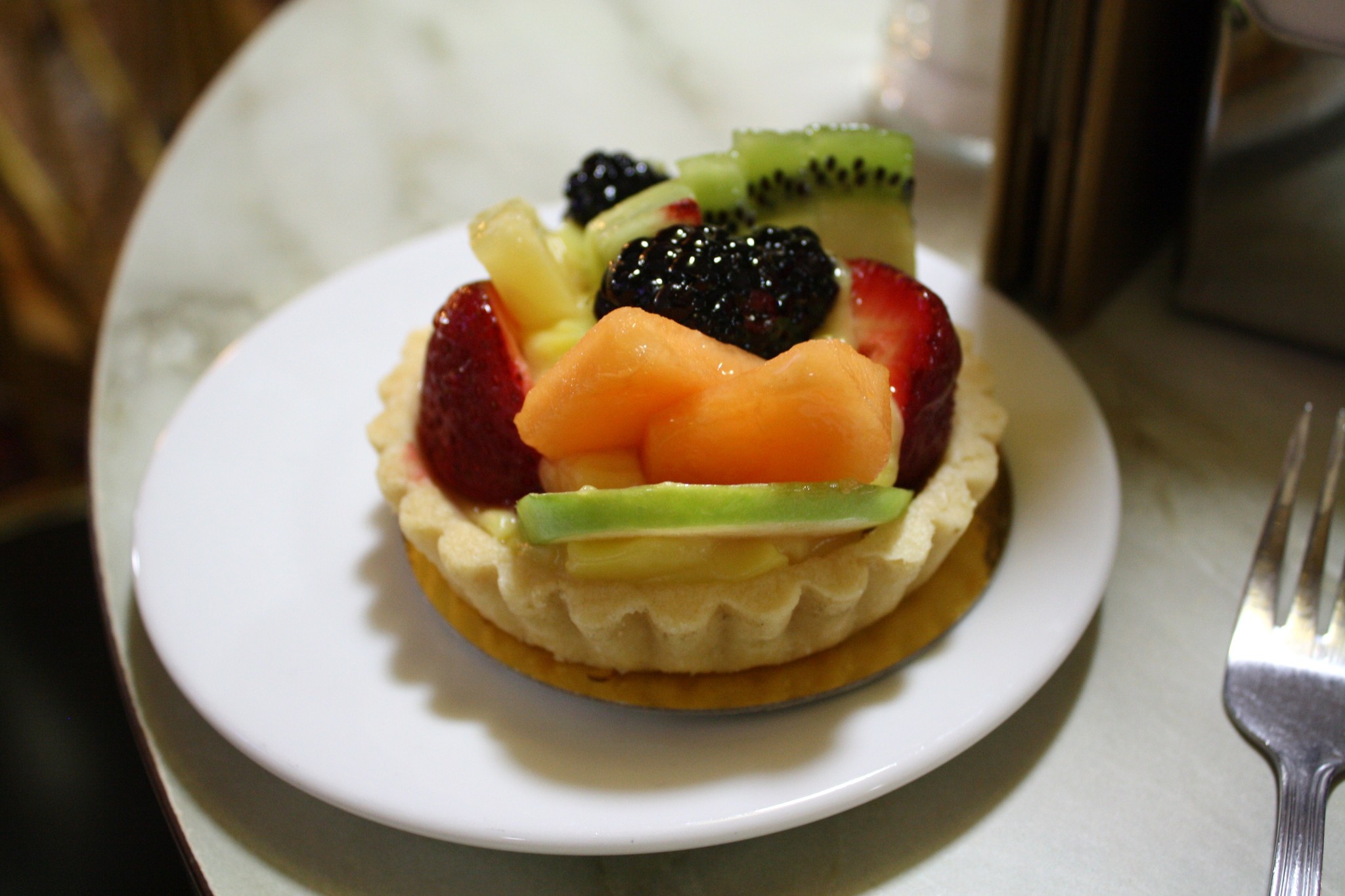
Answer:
[134,199,1120,860]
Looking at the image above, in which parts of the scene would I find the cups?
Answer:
[868,1,1010,168]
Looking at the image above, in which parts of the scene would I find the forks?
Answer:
[1223,399,1344,896]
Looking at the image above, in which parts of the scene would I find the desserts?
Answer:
[368,123,1010,714]
